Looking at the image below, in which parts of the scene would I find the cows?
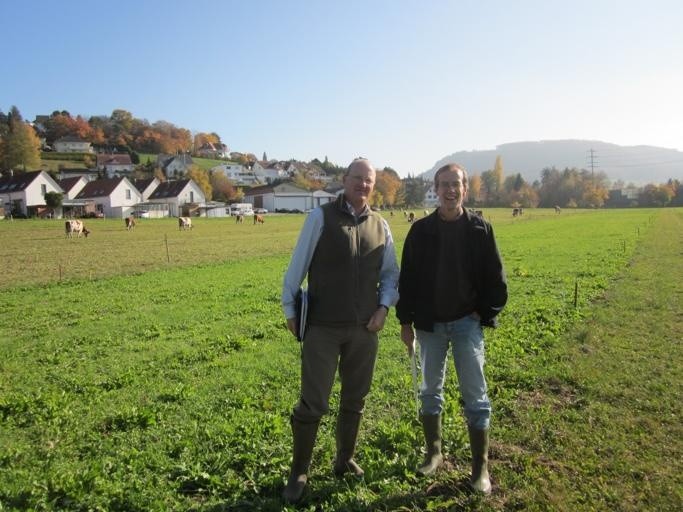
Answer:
[236,215,245,224]
[63,219,90,239]
[253,215,266,224]
[178,216,193,232]
[125,217,136,232]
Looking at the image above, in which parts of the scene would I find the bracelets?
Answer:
[378,303,389,313]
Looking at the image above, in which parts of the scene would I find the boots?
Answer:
[466,423,492,497]
[334,407,365,477]
[280,414,320,505]
[416,407,444,478]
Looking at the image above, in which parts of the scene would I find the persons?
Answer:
[280,158,401,505]
[395,163,509,495]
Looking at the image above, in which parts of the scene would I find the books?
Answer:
[294,286,310,343]
